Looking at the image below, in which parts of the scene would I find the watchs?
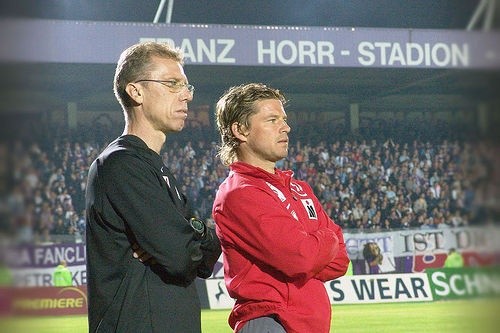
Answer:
[186,217,205,240]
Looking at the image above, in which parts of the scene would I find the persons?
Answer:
[0,118,499,286]
[212,83,349,333]
[85,42,221,333]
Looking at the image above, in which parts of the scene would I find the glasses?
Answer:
[135,79,194,93]
